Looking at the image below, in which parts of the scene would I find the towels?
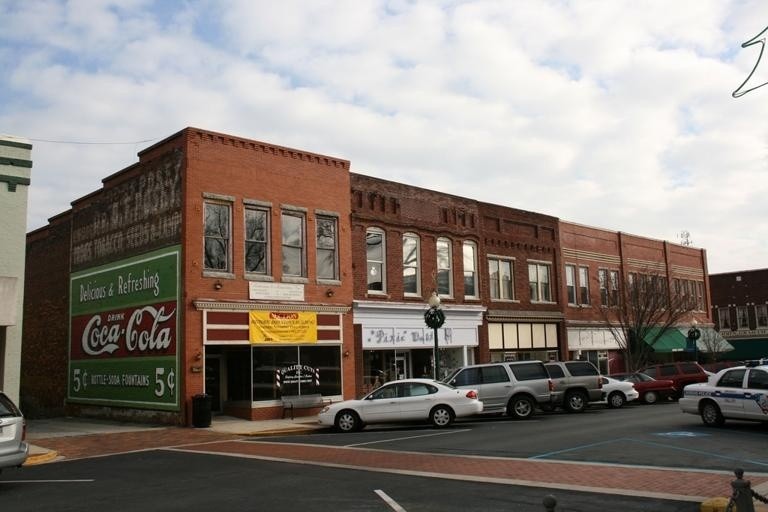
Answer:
[192,394,212,428]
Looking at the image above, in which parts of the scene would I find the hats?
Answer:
[601,375,639,408]
[315,379,484,433]
[677,364,768,427]
[0,392,28,469]
[613,372,677,405]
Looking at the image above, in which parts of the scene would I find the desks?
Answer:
[689,318,698,359]
[430,293,442,378]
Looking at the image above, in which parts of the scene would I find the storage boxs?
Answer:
[281,393,332,420]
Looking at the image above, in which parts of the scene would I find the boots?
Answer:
[442,361,554,418]
[545,361,604,412]
[637,362,709,402]
